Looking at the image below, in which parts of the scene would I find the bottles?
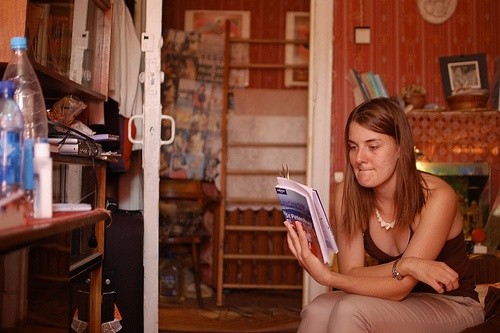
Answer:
[0,37,50,217]
[0,79,26,225]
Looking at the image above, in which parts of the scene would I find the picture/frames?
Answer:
[439,53,490,99]
[285,12,310,87]
[184,10,250,87]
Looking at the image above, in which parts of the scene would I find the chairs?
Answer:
[461,192,500,333]
[159,175,216,308]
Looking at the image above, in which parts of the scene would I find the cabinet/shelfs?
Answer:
[0,0,310,333]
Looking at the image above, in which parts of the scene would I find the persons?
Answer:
[189,83,207,129]
[282,96,486,333]
[453,66,469,87]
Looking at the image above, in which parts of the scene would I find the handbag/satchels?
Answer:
[164,210,210,238]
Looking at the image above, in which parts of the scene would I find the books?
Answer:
[344,68,390,101]
[90,133,119,141]
[274,164,339,265]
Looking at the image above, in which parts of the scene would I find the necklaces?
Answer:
[375,208,396,230]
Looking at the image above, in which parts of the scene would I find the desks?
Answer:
[405,109,500,207]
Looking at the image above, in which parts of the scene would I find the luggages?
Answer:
[69,207,145,333]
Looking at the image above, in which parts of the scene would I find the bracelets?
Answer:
[392,258,406,280]
